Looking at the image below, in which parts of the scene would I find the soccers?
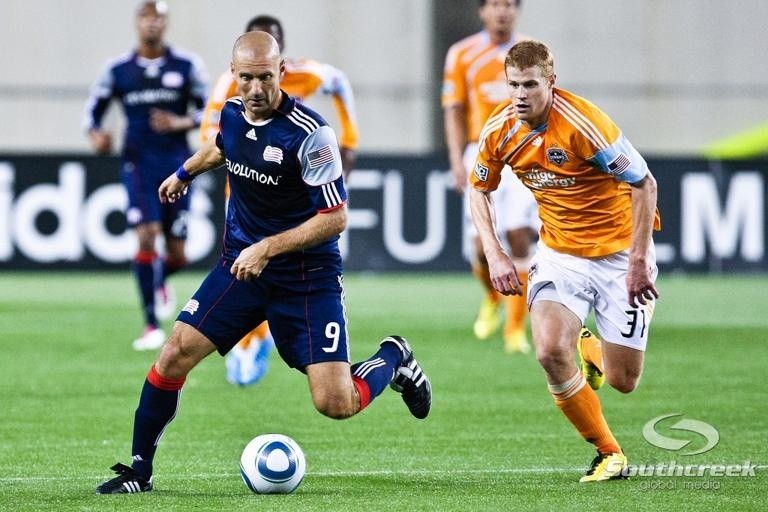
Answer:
[238,434,306,494]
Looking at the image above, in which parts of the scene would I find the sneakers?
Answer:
[472,293,505,340]
[503,320,527,354]
[132,328,165,350]
[576,325,605,390]
[155,285,174,319]
[95,463,153,494]
[240,335,272,384]
[379,336,432,419]
[224,347,242,386]
[579,448,630,483]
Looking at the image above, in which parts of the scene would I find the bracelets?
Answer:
[176,164,196,185]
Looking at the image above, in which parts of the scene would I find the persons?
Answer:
[92,31,431,495]
[82,1,208,360]
[199,14,357,386]
[438,0,533,356]
[466,39,662,486]
